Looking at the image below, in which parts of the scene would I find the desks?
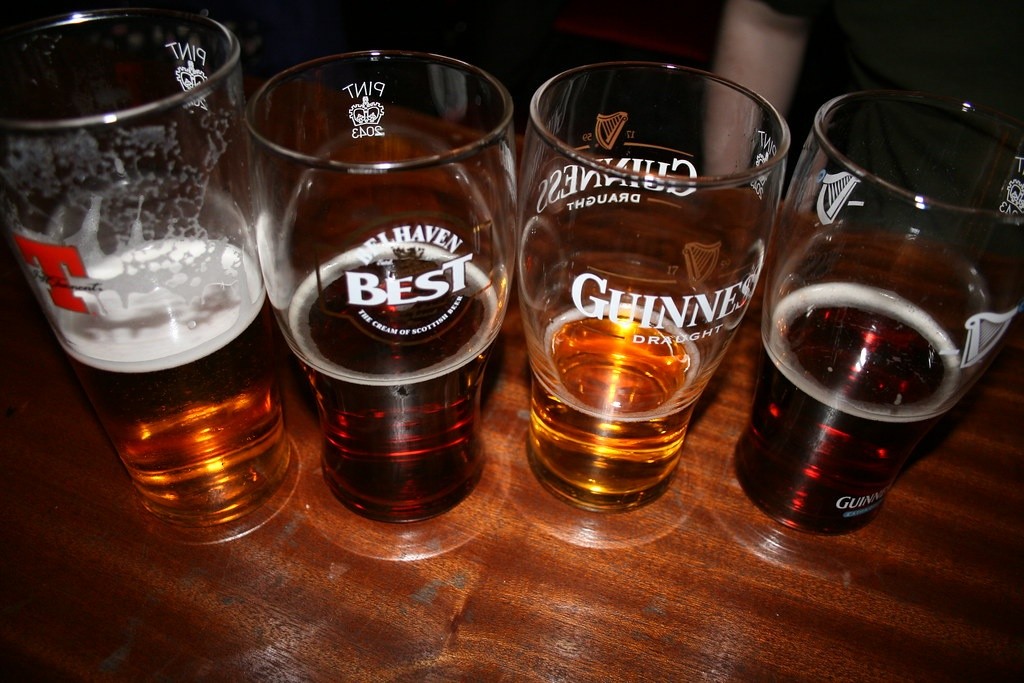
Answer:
[0,68,1024,683]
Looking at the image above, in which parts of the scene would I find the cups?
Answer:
[517,62,791,515]
[244,48,517,523]
[0,10,289,526]
[735,87,1024,535]
